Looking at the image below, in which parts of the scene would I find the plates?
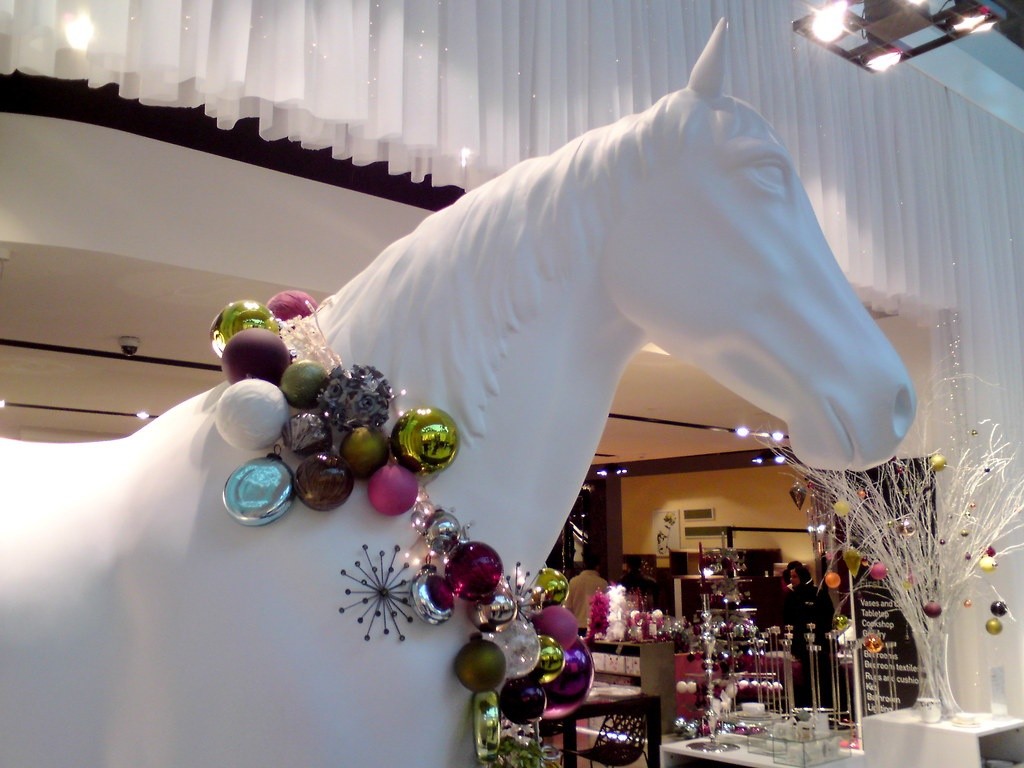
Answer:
[951,719,982,728]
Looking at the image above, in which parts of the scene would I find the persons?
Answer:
[616,554,659,606]
[562,551,609,641]
[748,561,847,710]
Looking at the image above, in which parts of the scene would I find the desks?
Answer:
[539,689,663,768]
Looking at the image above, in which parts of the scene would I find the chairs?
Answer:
[558,693,648,768]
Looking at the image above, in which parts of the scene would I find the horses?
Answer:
[2,15,920,768]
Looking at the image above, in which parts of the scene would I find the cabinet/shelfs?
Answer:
[660,707,1024,768]
[543,641,675,768]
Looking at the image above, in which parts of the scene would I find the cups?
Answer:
[814,713,829,734]
[917,698,941,722]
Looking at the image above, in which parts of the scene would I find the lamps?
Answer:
[793,0,1000,75]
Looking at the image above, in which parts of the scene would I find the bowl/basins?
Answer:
[955,712,976,725]
[742,702,765,713]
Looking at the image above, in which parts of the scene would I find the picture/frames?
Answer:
[653,509,681,558]
